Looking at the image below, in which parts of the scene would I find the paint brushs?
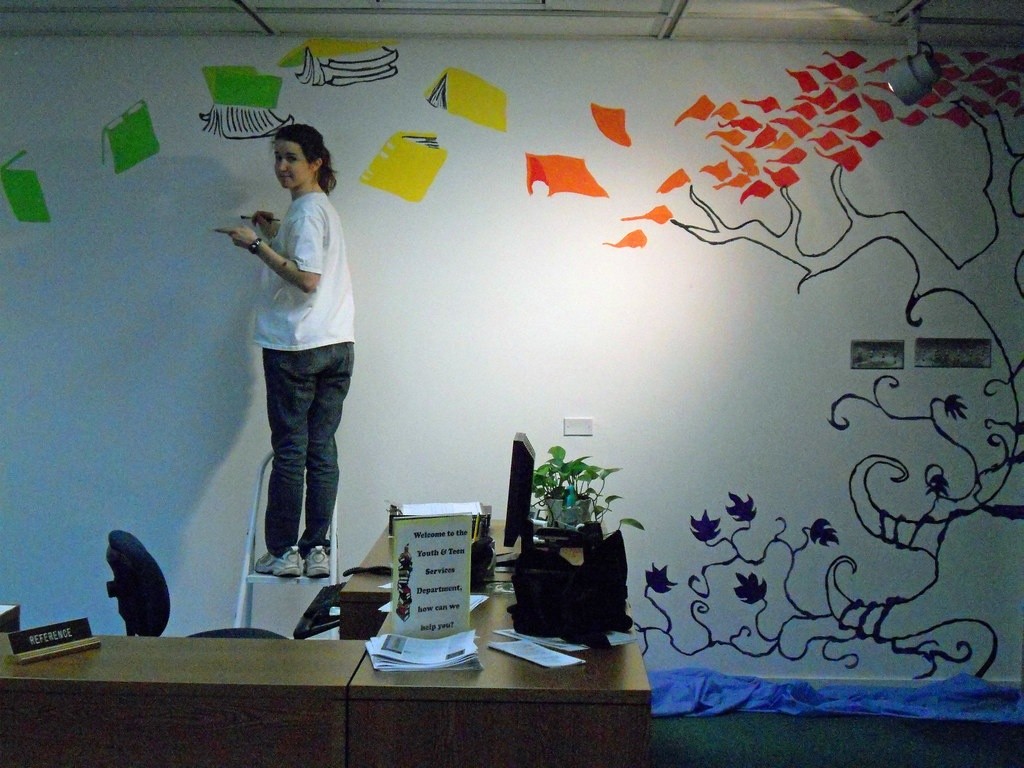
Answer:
[241,215,281,222]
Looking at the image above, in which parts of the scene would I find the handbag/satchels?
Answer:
[507,521,633,639]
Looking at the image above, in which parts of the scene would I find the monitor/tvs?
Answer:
[494,432,535,567]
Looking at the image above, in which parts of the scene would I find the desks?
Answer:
[0,632,368,768]
[340,520,652,768]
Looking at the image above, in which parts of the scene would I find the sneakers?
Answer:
[255,545,303,577]
[304,545,330,577]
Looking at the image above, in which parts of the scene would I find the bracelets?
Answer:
[245,238,262,255]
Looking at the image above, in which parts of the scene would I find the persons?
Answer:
[208,124,356,577]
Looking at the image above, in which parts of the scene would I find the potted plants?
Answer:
[530,446,645,536]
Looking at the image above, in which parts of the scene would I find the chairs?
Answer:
[106,530,290,640]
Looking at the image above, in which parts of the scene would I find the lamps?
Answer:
[887,10,941,106]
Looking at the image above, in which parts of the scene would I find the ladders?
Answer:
[230,450,342,642]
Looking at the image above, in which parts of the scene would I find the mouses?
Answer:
[311,606,341,624]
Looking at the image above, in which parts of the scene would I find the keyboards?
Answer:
[304,582,347,618]
